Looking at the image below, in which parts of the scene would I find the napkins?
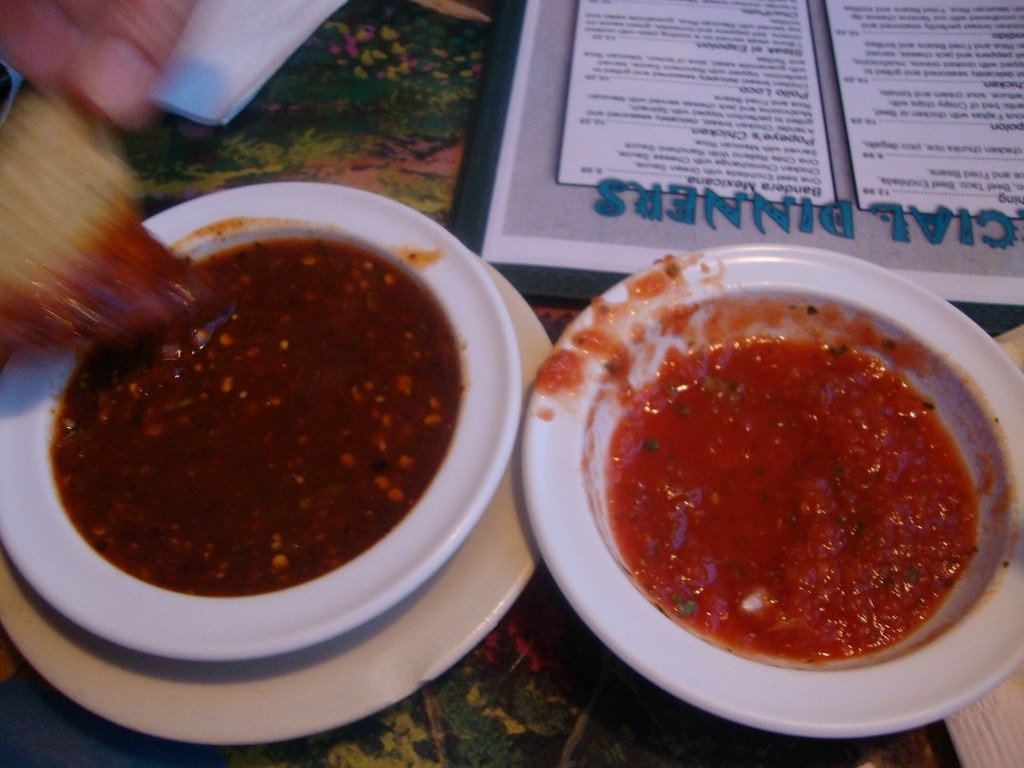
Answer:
[938,319,1023,768]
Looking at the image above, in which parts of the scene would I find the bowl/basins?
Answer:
[0,179,525,664]
[515,240,1024,739]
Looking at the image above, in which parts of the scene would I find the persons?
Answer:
[0,0,197,130]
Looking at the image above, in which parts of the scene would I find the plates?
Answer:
[1,250,558,748]
